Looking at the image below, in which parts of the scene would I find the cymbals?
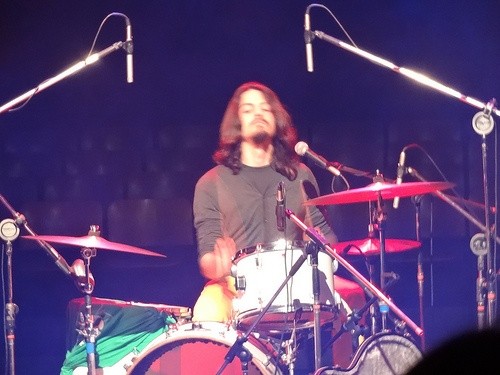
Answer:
[301,179,455,207]
[19,224,167,260]
[330,238,421,256]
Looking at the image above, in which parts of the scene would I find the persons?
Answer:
[192,82,340,364]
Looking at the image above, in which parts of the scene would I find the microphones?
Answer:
[127,20,134,83]
[305,13,314,73]
[274,185,287,232]
[393,152,405,209]
[294,141,342,176]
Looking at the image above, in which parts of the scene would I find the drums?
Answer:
[228,240,338,331]
[126,319,286,374]
